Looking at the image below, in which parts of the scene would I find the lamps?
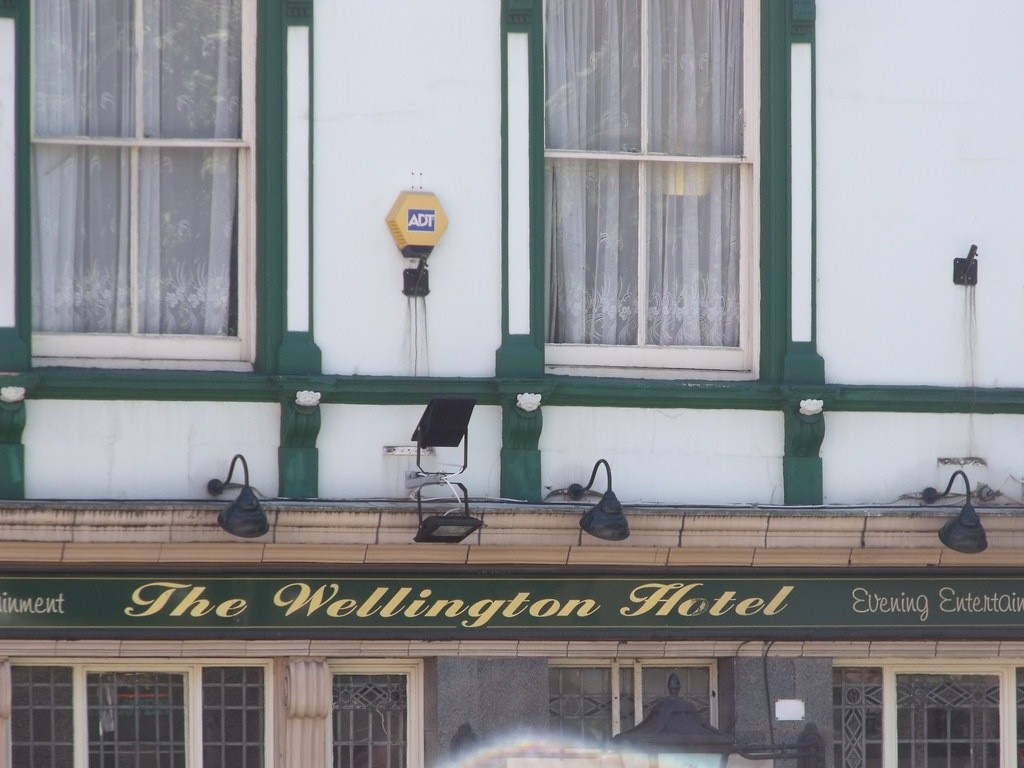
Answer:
[569,458,632,542]
[923,469,990,554]
[611,674,740,768]
[413,481,481,545]
[208,456,271,539]
[410,394,477,476]
